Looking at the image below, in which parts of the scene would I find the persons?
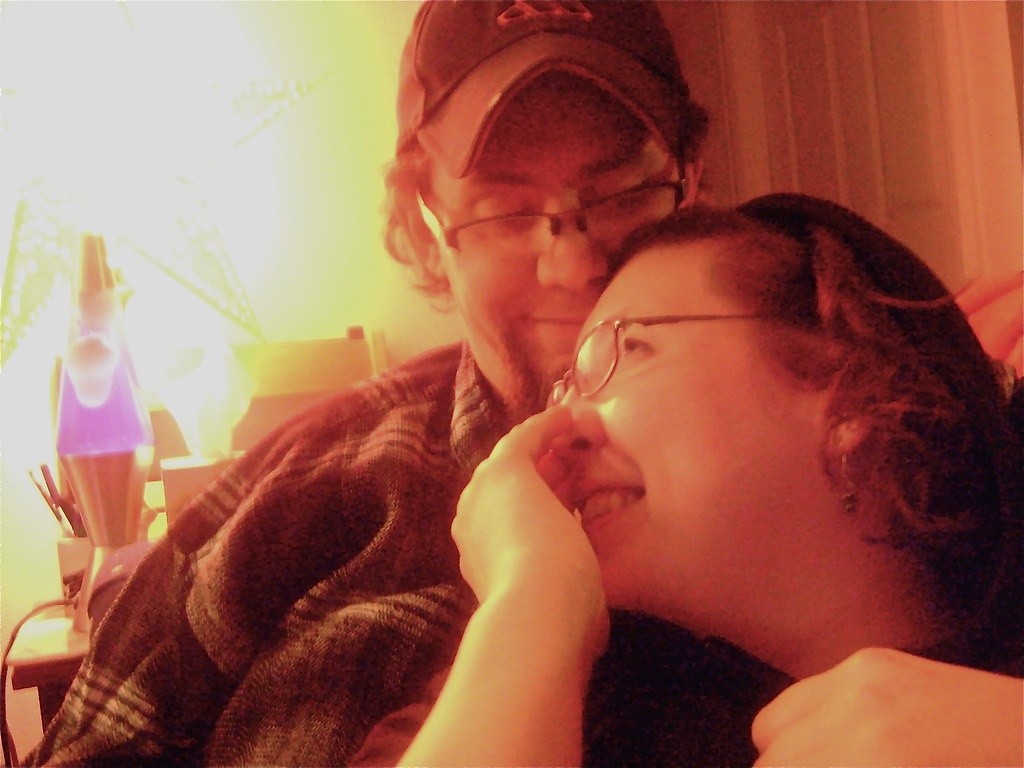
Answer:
[13,0,707,768]
[397,194,1024,768]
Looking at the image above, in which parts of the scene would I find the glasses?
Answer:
[544,313,824,413]
[417,145,688,285]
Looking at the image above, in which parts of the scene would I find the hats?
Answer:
[395,0,690,179]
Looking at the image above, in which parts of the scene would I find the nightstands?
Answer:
[8,616,95,737]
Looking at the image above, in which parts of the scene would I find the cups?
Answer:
[57,537,93,617]
